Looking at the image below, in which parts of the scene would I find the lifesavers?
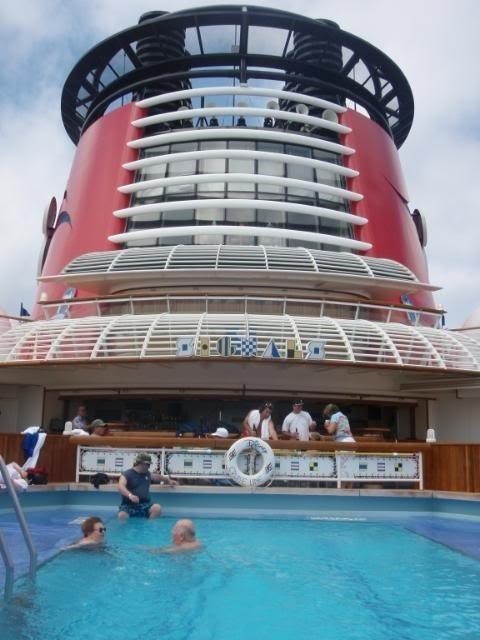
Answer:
[225,436,276,487]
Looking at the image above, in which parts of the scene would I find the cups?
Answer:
[325,419,330,424]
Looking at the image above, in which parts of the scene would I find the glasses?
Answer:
[93,527,107,533]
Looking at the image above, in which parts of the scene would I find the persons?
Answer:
[281,399,318,441]
[148,518,205,559]
[322,403,360,489]
[90,419,108,436]
[117,453,180,522]
[243,400,278,441]
[72,406,89,432]
[60,515,106,552]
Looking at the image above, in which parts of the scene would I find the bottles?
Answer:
[62,421,73,436]
[252,422,258,437]
[426,428,436,443]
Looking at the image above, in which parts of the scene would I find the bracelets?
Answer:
[128,493,132,498]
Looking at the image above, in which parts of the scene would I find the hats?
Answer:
[134,454,154,465]
[292,400,304,407]
[91,419,108,427]
